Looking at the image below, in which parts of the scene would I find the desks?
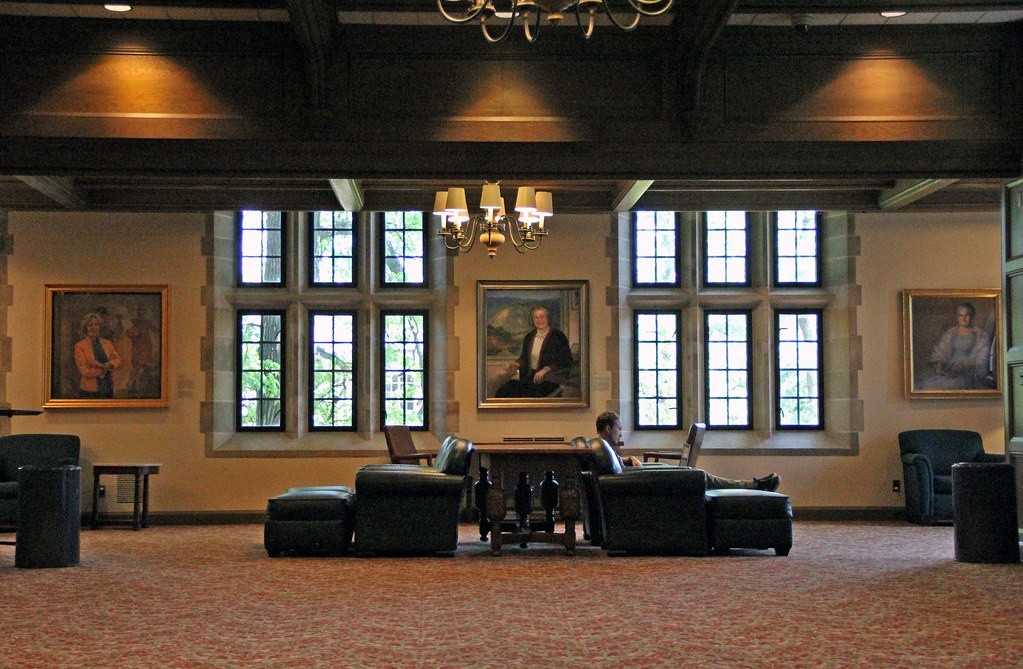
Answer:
[474,445,592,554]
[91,463,160,530]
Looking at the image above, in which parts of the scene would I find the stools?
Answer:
[705,489,793,557]
[265,486,356,559]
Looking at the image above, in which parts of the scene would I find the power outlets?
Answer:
[98,485,106,497]
[892,479,901,492]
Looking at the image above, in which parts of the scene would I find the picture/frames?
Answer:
[902,287,1003,400]
[475,277,590,409]
[41,283,171,410]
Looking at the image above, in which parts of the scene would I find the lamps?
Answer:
[438,0,671,43]
[434,181,553,255]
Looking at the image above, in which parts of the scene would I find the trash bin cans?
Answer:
[951,462,1021,564]
[15,465,81,567]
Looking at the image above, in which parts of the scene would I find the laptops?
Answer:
[679,443,691,467]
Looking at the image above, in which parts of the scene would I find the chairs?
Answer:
[383,423,442,466]
[643,422,707,467]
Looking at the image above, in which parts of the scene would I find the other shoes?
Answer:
[758,476,781,491]
[754,473,776,482]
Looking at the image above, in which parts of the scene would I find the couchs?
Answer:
[898,429,1003,526]
[351,436,473,555]
[573,437,707,559]
[0,434,80,533]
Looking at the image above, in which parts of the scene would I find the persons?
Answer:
[595,410,782,491]
